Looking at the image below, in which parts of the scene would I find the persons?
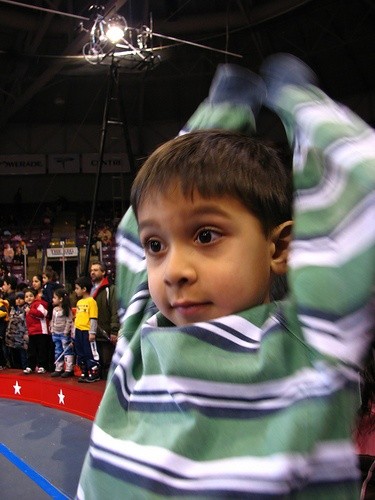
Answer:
[38,196,67,249]
[14,188,22,205]
[73,53,375,500]
[39,268,64,320]
[355,348,375,494]
[49,289,75,377]
[90,263,120,379]
[98,226,112,246]
[72,277,101,383]
[90,233,101,254]
[0,230,31,276]
[0,276,29,370]
[77,224,88,246]
[32,274,45,300]
[23,288,49,374]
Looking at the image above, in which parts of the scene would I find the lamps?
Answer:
[103,14,129,43]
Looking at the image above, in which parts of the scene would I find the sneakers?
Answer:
[37,367,46,373]
[78,373,89,381]
[85,373,100,382]
[23,367,32,373]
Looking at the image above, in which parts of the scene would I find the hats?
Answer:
[15,292,24,299]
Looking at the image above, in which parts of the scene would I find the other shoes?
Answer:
[61,371,74,377]
[51,370,65,376]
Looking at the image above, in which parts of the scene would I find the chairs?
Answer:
[4,223,115,281]
